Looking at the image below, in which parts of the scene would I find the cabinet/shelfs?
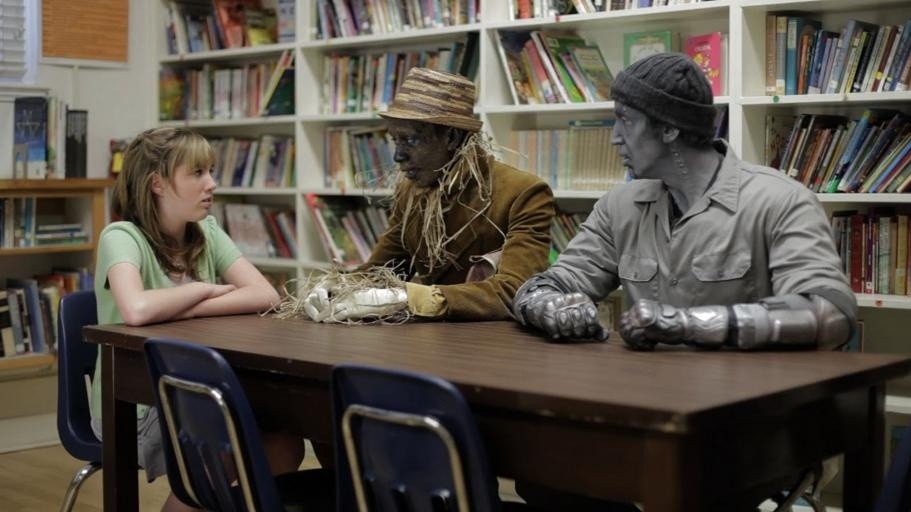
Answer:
[0,178,116,454]
[155,1,911,416]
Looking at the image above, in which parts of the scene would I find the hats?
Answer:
[609,52,716,136]
[380,65,483,135]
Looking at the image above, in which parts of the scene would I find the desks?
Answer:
[82,309,911,512]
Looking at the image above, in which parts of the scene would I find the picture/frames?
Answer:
[837,320,865,352]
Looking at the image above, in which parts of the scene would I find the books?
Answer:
[622,29,728,98]
[763,109,911,193]
[0,265,94,356]
[212,199,296,262]
[207,134,295,190]
[308,194,391,266]
[827,209,911,297]
[712,103,728,145]
[321,33,480,114]
[13,96,89,180]
[494,31,614,105]
[155,51,296,120]
[163,2,296,52]
[323,124,406,191]
[313,1,483,40]
[513,0,710,19]
[509,118,631,189]
[0,196,88,248]
[763,11,911,95]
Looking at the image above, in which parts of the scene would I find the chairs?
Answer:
[144,338,337,511]
[331,363,527,512]
[56,289,102,512]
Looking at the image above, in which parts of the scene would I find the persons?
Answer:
[304,67,555,319]
[514,54,857,357]
[92,125,305,511]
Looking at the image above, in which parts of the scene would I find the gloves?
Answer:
[515,286,610,343]
[314,287,407,323]
[305,278,344,320]
[620,291,817,350]
